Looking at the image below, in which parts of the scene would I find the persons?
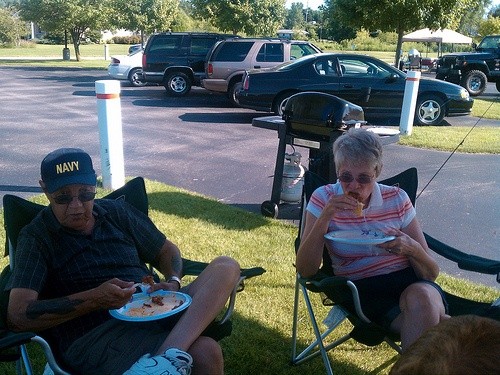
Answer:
[295,127,453,356]
[4,148,240,375]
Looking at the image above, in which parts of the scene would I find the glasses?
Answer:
[336,166,377,184]
[46,190,95,205]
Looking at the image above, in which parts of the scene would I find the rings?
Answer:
[389,248,391,253]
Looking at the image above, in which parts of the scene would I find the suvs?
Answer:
[435,36,500,94]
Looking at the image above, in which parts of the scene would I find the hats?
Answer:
[41,147,96,194]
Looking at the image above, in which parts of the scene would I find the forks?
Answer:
[123,283,151,291]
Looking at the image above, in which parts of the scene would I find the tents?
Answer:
[401,27,472,57]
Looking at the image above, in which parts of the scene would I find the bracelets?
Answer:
[167,277,181,290]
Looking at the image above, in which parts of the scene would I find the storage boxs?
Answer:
[422,58,432,65]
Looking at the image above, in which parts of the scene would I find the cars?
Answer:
[231,52,473,126]
[108,48,164,88]
[141,31,242,96]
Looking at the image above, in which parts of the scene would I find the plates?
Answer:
[323,230,398,244]
[108,291,193,321]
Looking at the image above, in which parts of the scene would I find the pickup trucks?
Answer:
[201,40,372,112]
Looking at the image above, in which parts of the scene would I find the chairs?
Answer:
[0,176,267,375]
[290,170,500,375]
[341,64,346,73]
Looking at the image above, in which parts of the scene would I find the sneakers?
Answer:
[122,347,192,375]
